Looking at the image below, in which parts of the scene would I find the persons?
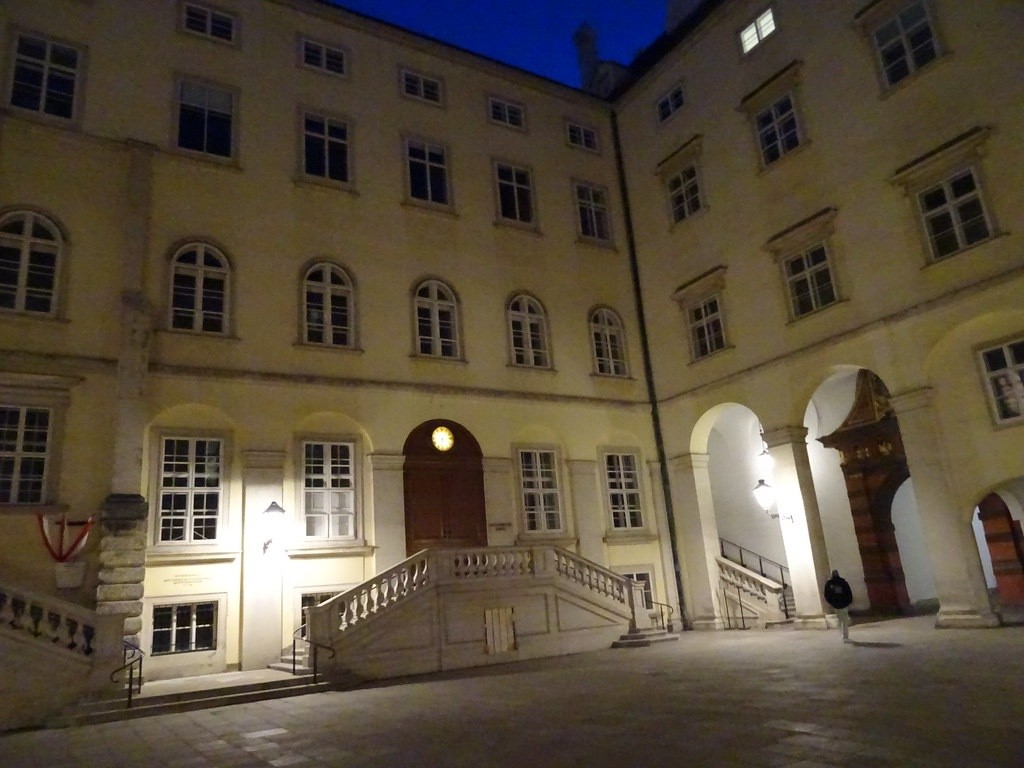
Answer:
[823,570,855,643]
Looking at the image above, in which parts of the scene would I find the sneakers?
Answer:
[843,638,853,642]
